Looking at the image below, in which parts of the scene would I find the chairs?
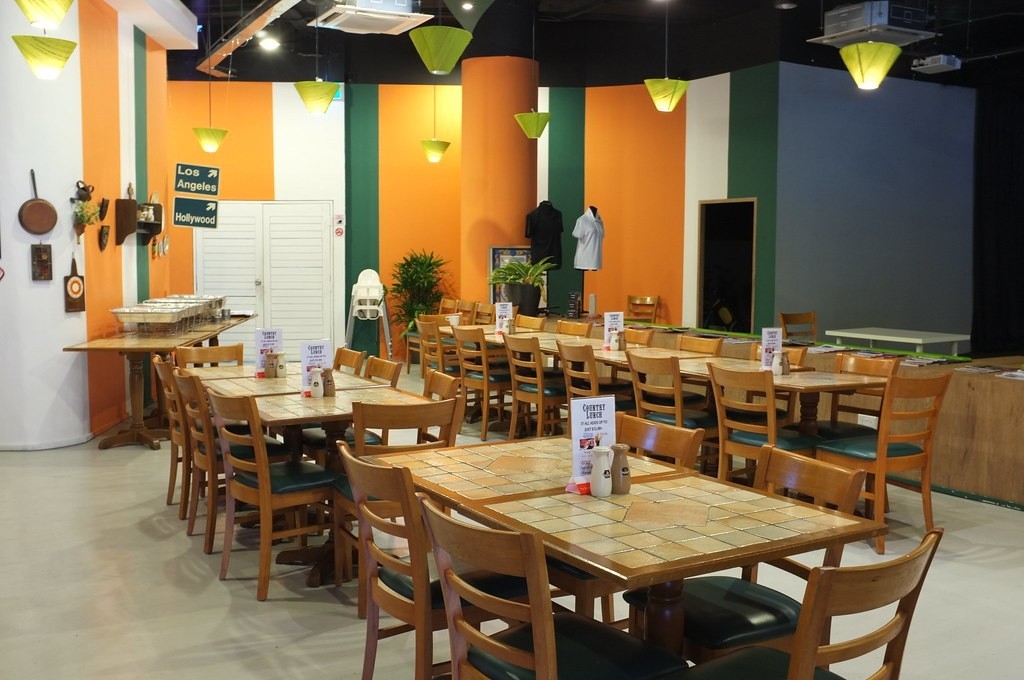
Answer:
[154,295,957,679]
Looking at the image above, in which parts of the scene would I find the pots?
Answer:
[18,169,57,234]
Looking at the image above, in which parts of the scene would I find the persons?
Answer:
[572,206,604,271]
[524,201,563,271]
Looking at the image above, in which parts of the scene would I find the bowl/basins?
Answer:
[140,212,148,221]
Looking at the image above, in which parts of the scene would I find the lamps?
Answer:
[294,0,340,114]
[15,0,73,29]
[420,76,450,163]
[407,0,473,77]
[11,35,77,80]
[641,0,690,112]
[255,29,282,52]
[514,8,553,138]
[837,40,902,90]
[192,0,228,153]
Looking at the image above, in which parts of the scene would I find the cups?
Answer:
[446,316,460,326]
[163,235,169,254]
[158,242,163,256]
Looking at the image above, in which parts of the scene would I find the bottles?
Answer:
[275,352,287,378]
[265,353,275,378]
[501,318,516,335]
[323,368,335,397]
[311,369,324,398]
[147,206,154,222]
[609,330,619,351]
[781,351,790,375]
[610,443,632,494]
[590,447,612,496]
[619,331,626,351]
[771,351,783,375]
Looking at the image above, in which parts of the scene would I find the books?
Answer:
[569,320,1024,381]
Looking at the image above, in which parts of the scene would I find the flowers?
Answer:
[75,202,105,224]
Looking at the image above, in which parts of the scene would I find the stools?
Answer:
[345,268,391,360]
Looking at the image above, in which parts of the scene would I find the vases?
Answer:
[74,222,86,243]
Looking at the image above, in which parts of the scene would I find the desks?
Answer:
[63,312,970,657]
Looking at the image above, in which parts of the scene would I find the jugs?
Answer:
[70,180,94,203]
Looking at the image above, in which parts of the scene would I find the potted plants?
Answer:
[391,250,442,364]
[483,255,559,318]
